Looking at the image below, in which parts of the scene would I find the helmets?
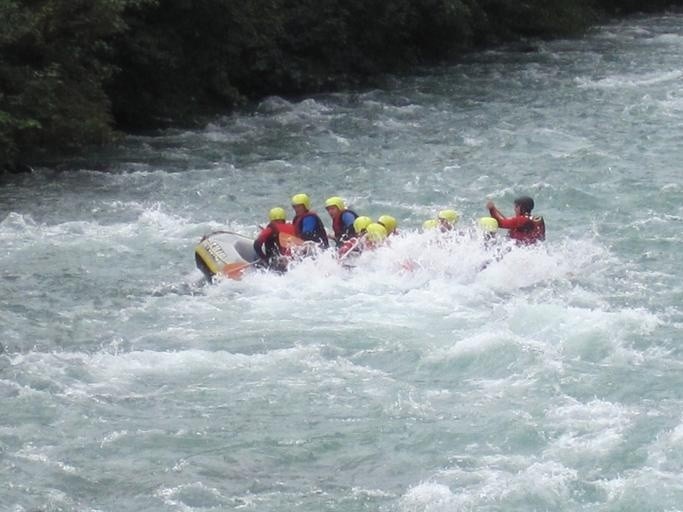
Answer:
[366,223,387,243]
[378,214,396,232]
[353,216,373,235]
[480,217,499,234]
[513,196,534,211]
[268,207,286,220]
[291,193,311,210]
[423,220,439,230]
[324,197,346,211]
[439,210,460,225]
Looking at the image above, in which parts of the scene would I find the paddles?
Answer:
[224,259,260,280]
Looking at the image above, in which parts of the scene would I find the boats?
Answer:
[195,232,509,293]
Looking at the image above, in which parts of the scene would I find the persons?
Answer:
[485,196,545,246]
[290,194,329,251]
[324,197,499,258]
[253,207,304,272]
